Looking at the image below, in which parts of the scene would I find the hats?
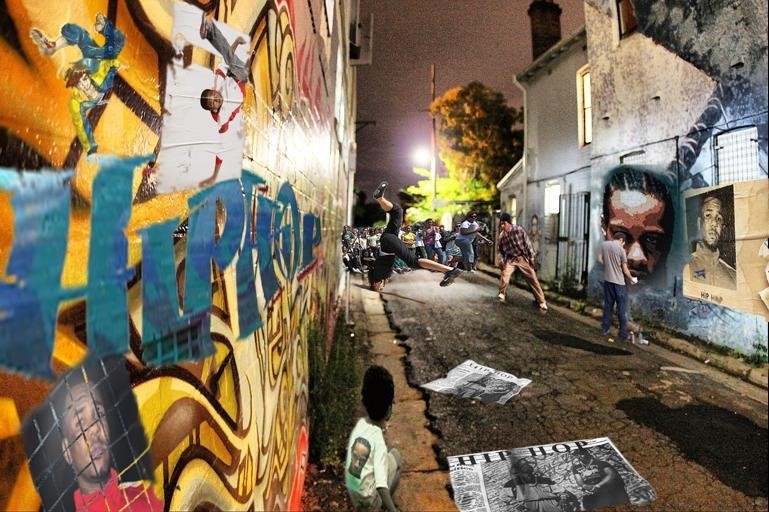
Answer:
[500,212,512,224]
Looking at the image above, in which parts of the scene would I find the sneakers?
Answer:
[539,301,547,309]
[373,181,388,200]
[439,267,463,287]
[498,293,506,299]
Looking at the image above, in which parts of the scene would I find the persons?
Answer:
[572,449,630,508]
[342,211,494,274]
[526,215,543,262]
[499,458,579,512]
[497,213,548,310]
[368,181,463,292]
[199,11,249,188]
[344,365,402,512]
[61,381,164,512]
[348,437,371,478]
[31,12,129,165]
[683,191,737,291]
[599,168,675,295]
[597,232,632,340]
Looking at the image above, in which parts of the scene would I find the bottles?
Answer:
[638,332,643,344]
[629,330,634,344]
